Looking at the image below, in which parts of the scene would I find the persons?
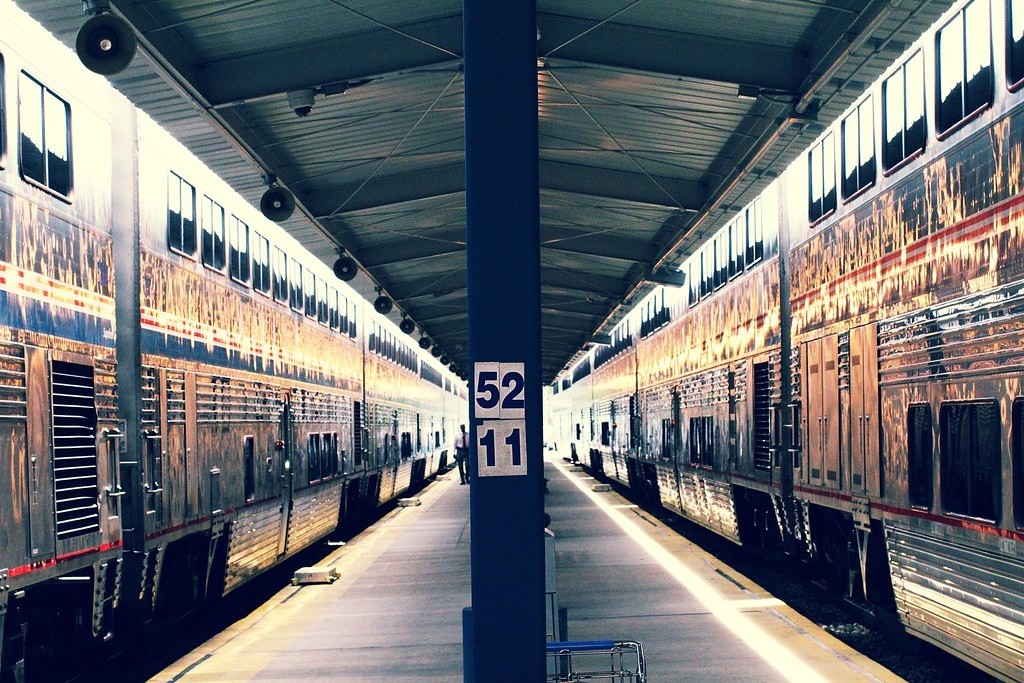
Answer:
[454,424,470,485]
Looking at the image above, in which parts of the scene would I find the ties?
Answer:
[462,433,466,449]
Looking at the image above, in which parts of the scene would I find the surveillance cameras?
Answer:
[287,88,315,117]
[643,267,686,288]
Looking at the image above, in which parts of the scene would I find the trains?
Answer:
[2,44,470,682]
[553,0,1024,683]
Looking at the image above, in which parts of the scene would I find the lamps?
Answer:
[259,174,296,223]
[400,312,415,334]
[75,0,137,77]
[431,342,469,388]
[419,330,431,350]
[373,285,392,314]
[333,246,358,281]
